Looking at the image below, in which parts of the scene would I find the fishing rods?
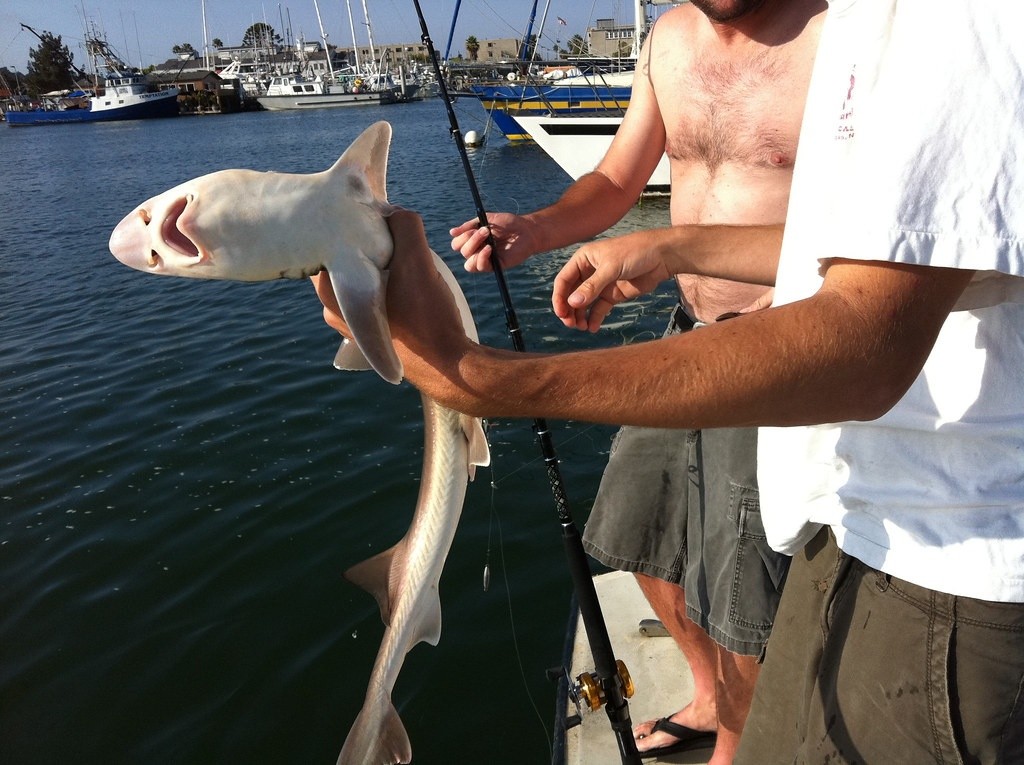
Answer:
[413,0,646,765]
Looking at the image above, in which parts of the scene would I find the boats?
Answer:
[494,63,671,197]
[4,38,181,126]
[247,72,420,114]
[469,62,634,143]
[547,568,715,763]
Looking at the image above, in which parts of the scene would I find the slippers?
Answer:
[636,716,717,758]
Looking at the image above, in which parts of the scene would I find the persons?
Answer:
[299,2,1023,765]
[447,0,829,765]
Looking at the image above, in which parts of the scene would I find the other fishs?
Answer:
[108,120,492,765]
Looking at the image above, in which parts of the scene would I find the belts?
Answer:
[673,308,692,333]
